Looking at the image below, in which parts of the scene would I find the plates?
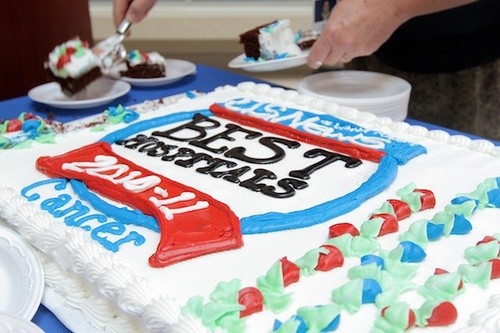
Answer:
[27,77,131,109]
[0,313,45,333]
[228,20,328,73]
[0,225,45,322]
[297,69,411,121]
[112,57,196,87]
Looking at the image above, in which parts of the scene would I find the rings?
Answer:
[315,61,322,65]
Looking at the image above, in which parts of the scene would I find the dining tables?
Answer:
[0,63,500,333]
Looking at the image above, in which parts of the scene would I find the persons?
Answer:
[113,0,500,142]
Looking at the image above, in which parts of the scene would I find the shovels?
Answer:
[93,18,132,74]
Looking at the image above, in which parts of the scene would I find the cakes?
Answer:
[115,49,168,78]
[239,18,320,63]
[0,79,500,332]
[44,36,103,97]
